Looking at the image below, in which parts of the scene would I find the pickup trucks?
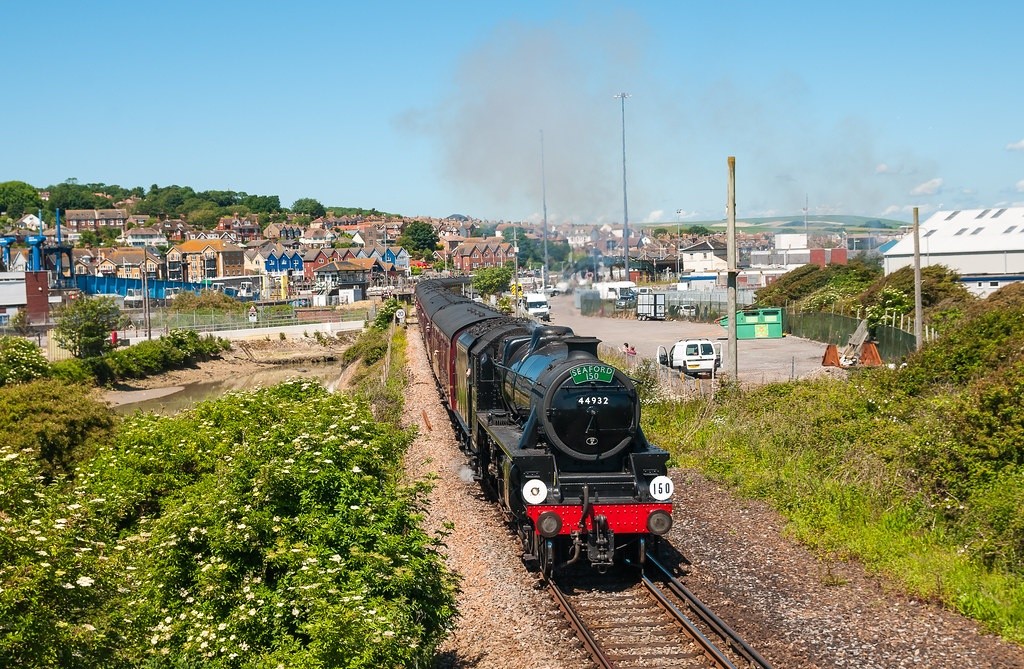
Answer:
[534,284,562,297]
[615,295,637,309]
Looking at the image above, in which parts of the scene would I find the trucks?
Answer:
[521,293,551,322]
[591,280,638,301]
[639,288,652,295]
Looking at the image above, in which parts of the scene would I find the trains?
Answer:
[413,275,674,583]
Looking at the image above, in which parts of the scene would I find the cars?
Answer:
[288,298,312,308]
[674,304,696,318]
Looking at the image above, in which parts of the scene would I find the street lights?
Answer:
[538,127,552,286]
[612,90,632,279]
[676,206,682,274]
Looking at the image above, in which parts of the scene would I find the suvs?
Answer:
[655,336,723,378]
